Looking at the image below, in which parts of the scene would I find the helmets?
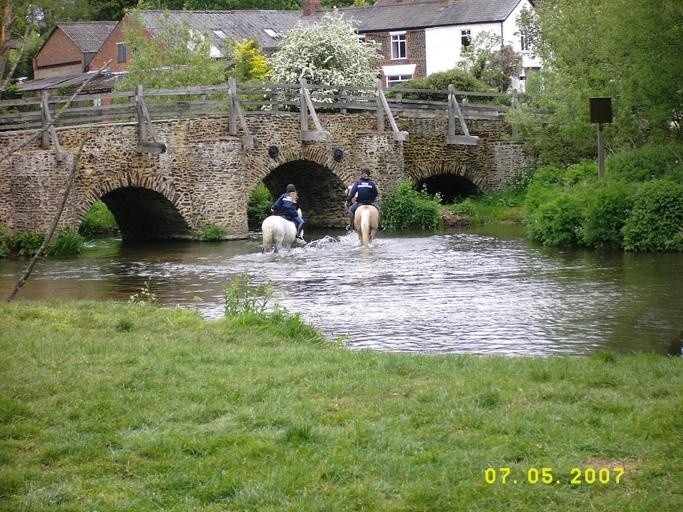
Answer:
[285,184,296,192]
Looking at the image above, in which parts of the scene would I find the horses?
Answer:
[261,207,304,255]
[344,183,380,249]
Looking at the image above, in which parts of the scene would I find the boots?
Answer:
[295,221,304,240]
[346,210,354,230]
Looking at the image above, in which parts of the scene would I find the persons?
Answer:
[271,183,305,240]
[346,168,385,229]
